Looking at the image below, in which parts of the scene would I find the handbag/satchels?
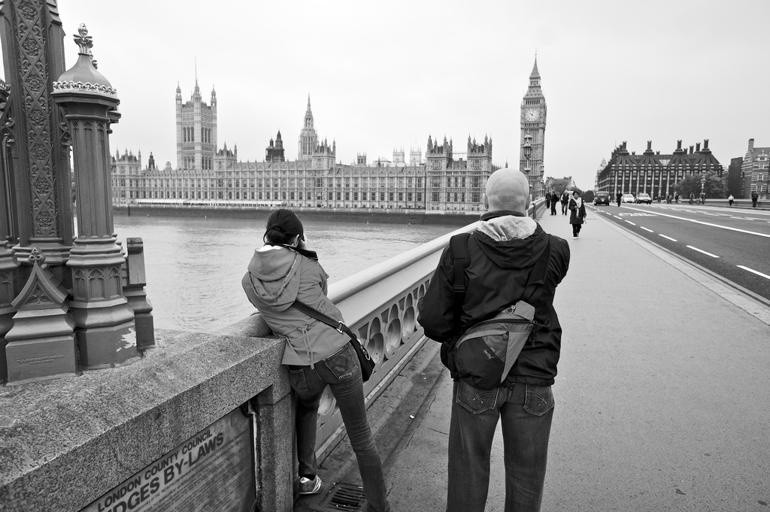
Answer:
[351,334,375,382]
[453,298,537,389]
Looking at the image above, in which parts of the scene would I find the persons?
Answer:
[656,191,706,205]
[616,189,622,207]
[569,191,587,237]
[550,191,559,215]
[241,209,390,511]
[546,191,551,209]
[728,193,734,206]
[418,169,570,512]
[560,190,569,215]
[752,190,758,207]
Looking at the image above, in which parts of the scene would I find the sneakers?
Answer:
[299,474,324,494]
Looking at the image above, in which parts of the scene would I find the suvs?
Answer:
[593,191,611,206]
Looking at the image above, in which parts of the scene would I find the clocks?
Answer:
[525,107,540,122]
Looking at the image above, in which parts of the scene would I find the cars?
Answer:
[636,193,652,204]
[621,194,635,203]
